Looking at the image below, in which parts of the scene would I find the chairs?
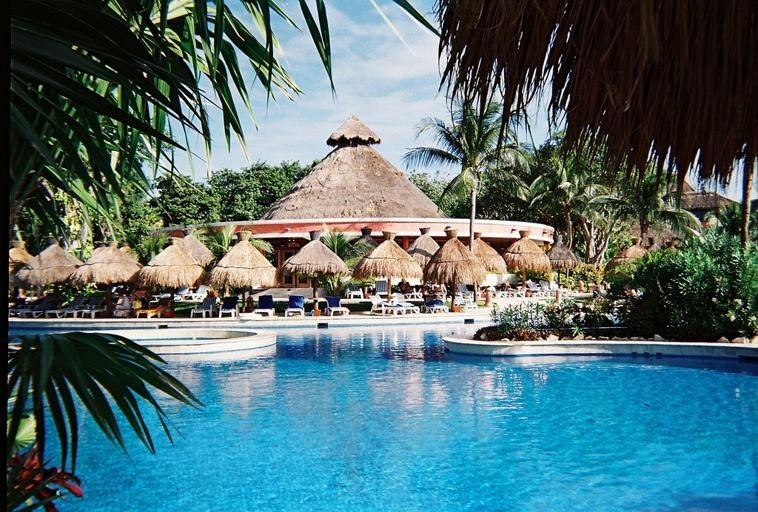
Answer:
[344,278,571,316]
[8,293,350,318]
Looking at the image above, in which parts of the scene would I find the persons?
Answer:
[111,289,150,311]
[206,288,224,308]
